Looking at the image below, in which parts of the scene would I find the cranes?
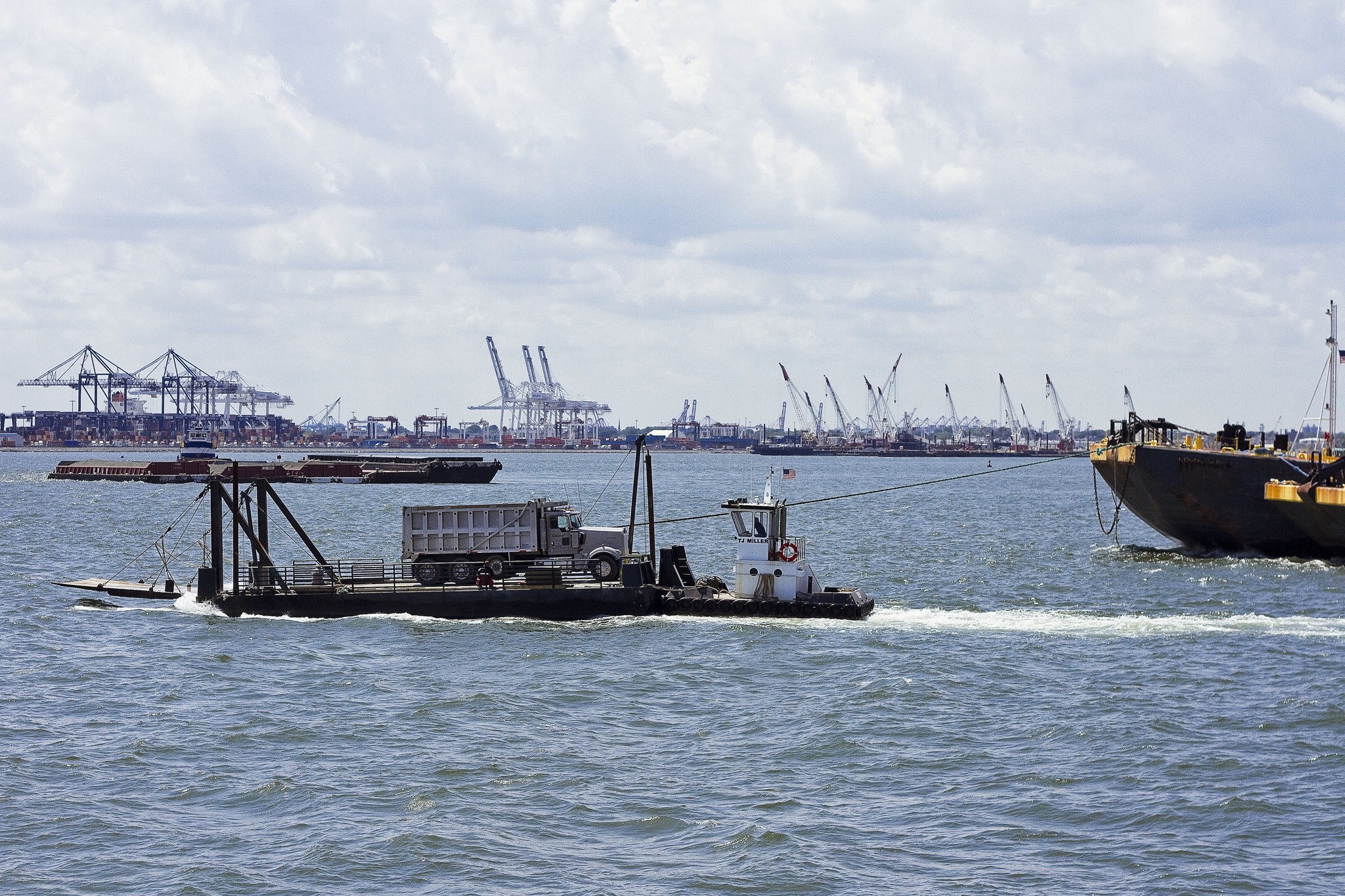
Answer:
[310,397,340,434]
[17,345,293,417]
[467,337,613,437]
[671,399,699,441]
[778,353,1075,451]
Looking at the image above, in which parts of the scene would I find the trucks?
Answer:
[399,496,626,581]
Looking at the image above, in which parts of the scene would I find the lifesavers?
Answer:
[780,542,798,560]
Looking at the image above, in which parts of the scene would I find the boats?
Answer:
[1089,300,1345,562]
[177,414,219,463]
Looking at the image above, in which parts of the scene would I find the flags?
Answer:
[783,469,796,479]
[1339,350,1345,363]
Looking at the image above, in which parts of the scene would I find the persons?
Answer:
[755,517,766,534]
[477,561,494,588]
[311,564,324,583]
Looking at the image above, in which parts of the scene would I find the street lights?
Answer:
[660,422,663,435]
[21,406,27,439]
[745,418,751,439]
[567,418,569,441]
[143,407,147,435]
[635,419,638,439]
[509,419,511,433]
[435,408,438,441]
[351,411,356,420]
[324,405,330,440]
[69,400,75,441]
[441,413,446,437]
